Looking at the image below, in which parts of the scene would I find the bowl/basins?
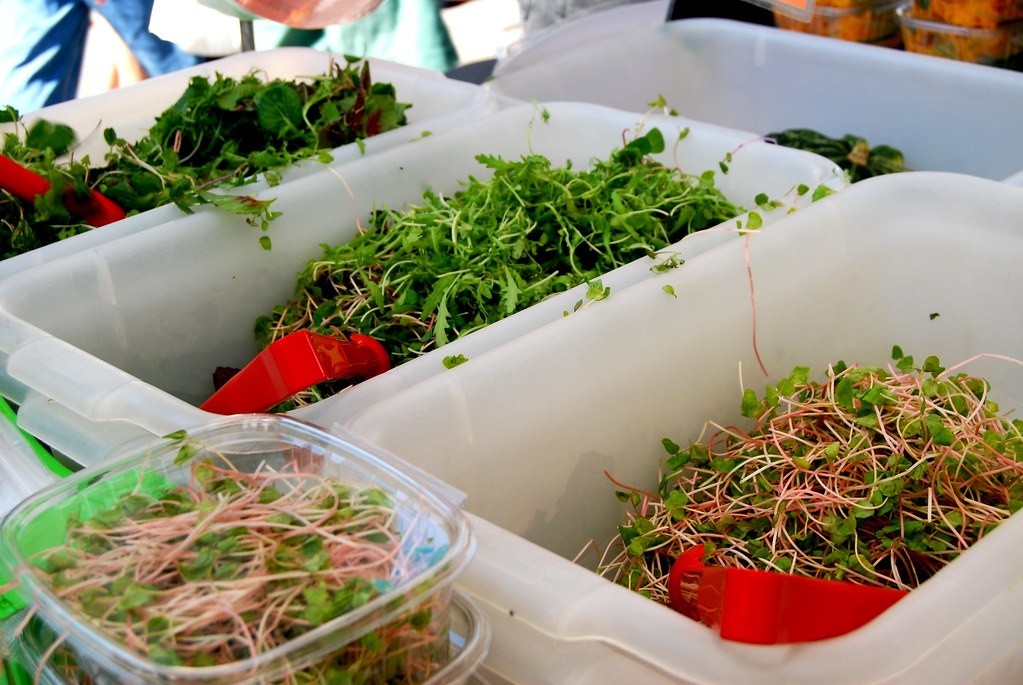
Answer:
[892,1,1023,69]
[2,413,482,685]
[2,588,493,685]
[771,1,906,49]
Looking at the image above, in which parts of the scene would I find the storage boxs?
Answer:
[4,16,1023,684]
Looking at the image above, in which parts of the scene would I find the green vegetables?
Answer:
[0,50,1022,685]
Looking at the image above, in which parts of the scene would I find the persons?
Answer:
[5,2,458,113]
